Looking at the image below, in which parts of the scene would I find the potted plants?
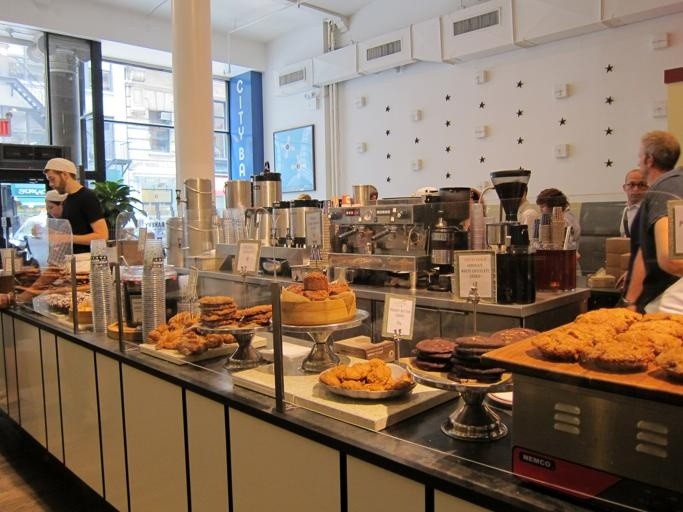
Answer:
[88,176,146,242]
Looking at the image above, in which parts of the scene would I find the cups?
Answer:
[139,240,166,342]
[138,228,147,250]
[0,247,15,270]
[325,264,354,285]
[532,205,566,246]
[175,275,198,315]
[212,209,245,248]
[468,203,499,251]
[89,239,112,333]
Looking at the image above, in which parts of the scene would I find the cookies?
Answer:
[416,329,540,383]
[320,358,411,390]
[199,296,273,329]
[532,307,683,376]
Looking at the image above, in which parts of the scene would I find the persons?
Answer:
[618,132,683,310]
[32,191,67,245]
[518,188,537,241]
[536,187,580,238]
[621,170,651,236]
[44,158,110,255]
[464,188,486,228]
[637,165,683,314]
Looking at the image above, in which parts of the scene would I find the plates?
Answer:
[317,361,415,402]
[486,391,512,406]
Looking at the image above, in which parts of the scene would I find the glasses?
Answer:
[626,182,647,188]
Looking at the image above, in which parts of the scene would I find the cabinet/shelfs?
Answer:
[276,287,371,347]
[371,299,587,360]
[194,276,285,312]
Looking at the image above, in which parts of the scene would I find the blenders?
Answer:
[490,167,530,250]
[440,188,472,225]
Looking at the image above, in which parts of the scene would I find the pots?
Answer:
[351,184,377,206]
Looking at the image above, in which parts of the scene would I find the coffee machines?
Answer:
[325,196,466,290]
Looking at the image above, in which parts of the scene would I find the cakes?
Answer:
[33,291,93,323]
[120,265,179,295]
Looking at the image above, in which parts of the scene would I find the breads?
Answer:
[280,271,356,325]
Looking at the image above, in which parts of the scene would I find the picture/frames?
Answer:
[271,124,317,194]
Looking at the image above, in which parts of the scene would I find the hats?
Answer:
[45,157,77,174]
[45,189,69,201]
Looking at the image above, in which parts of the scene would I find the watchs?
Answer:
[622,298,637,306]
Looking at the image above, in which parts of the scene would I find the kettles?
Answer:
[424,207,462,272]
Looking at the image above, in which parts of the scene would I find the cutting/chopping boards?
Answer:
[480,320,682,407]
[137,335,267,365]
[229,350,460,432]
